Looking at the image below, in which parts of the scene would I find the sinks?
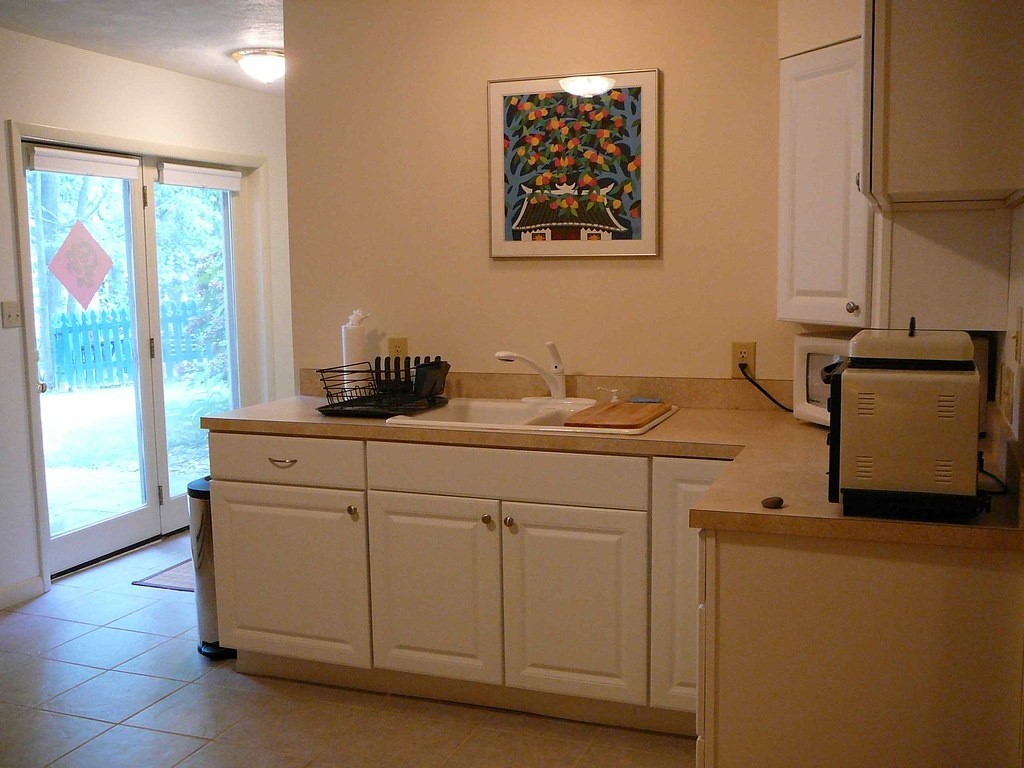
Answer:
[526,409,663,430]
[385,397,539,424]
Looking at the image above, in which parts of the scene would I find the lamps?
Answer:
[229,46,286,84]
[558,75,615,98]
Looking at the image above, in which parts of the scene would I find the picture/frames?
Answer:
[486,68,663,258]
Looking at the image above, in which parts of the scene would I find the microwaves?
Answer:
[792,332,849,428]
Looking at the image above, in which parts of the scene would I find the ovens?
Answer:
[822,317,981,523]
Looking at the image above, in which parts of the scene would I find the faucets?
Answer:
[493,341,567,398]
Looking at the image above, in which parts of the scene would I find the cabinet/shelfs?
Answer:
[696,527,1024,768]
[648,456,735,714]
[207,432,373,671]
[365,439,650,707]
[776,0,1012,331]
[860,0,1024,212]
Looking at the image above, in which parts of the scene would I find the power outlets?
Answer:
[388,338,407,360]
[731,341,756,379]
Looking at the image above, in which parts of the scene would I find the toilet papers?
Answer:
[341,309,371,400]
